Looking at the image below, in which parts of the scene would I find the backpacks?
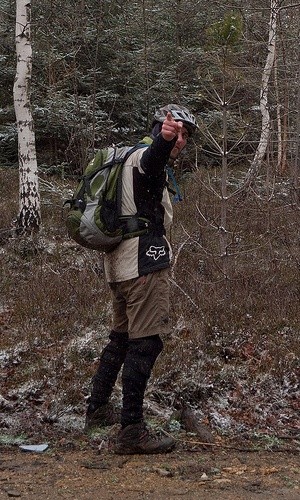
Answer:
[61,141,184,254]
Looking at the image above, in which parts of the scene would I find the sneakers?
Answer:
[114,420,176,456]
[84,401,121,428]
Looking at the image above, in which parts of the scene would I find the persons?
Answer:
[85,103,200,454]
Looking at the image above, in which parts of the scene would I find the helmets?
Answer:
[151,103,200,131]
